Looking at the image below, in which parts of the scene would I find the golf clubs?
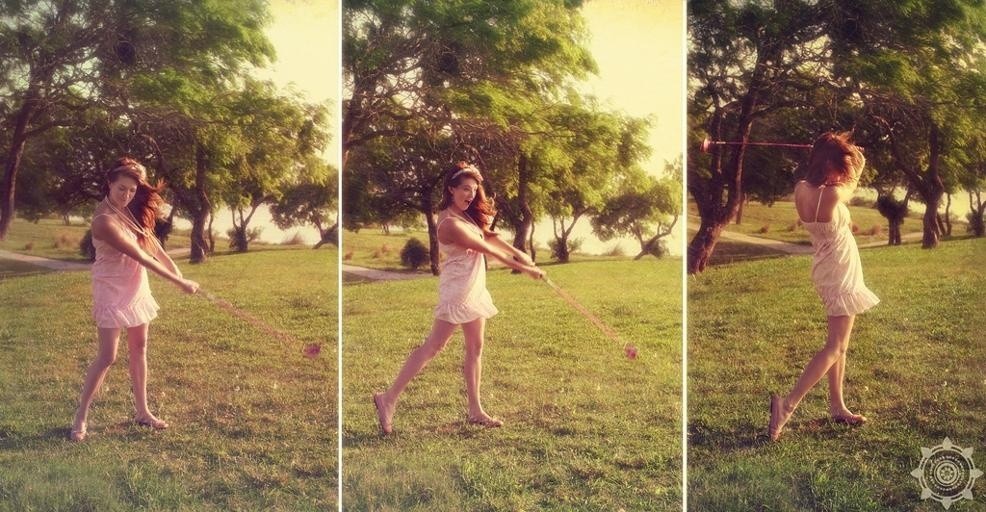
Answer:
[700,138,865,154]
[190,287,322,360]
[530,265,638,361]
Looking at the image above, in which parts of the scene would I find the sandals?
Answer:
[71,413,86,441]
[467,413,503,428]
[830,414,867,426]
[768,394,780,444]
[374,392,393,433]
[134,413,169,429]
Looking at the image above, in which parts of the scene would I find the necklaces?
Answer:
[104,194,150,237]
[448,207,479,227]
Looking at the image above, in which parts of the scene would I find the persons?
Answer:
[765,129,881,442]
[67,157,199,442]
[370,162,547,434]
